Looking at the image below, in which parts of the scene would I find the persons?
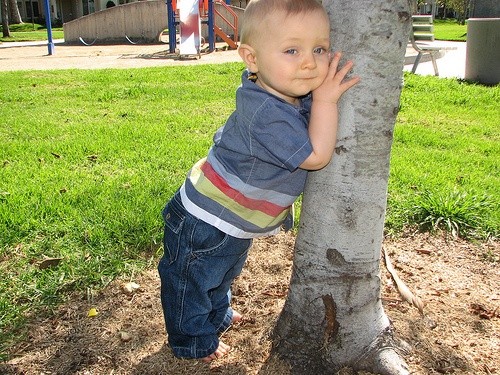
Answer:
[157,0,362,362]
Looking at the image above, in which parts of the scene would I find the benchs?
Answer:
[408,15,457,77]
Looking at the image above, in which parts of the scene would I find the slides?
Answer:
[177,0,202,60]
